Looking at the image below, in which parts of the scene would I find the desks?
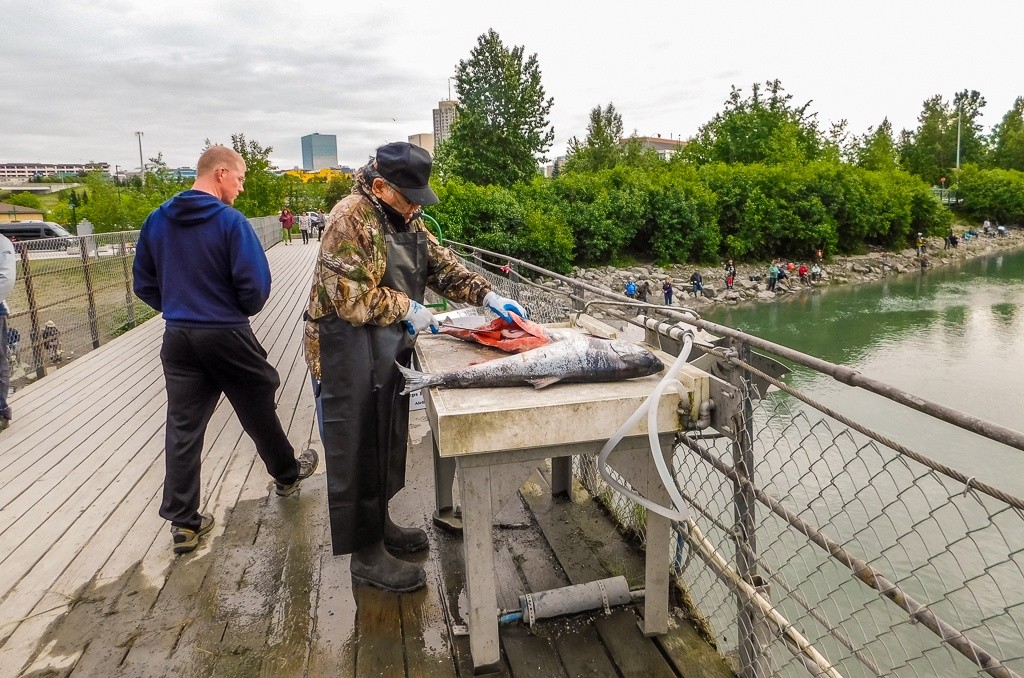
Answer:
[411,321,710,668]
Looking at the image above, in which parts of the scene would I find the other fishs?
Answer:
[424,309,573,352]
[394,334,666,394]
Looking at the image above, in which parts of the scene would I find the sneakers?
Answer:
[274,449,320,497]
[170,512,215,553]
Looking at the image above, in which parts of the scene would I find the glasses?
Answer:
[215,168,245,184]
[382,180,411,204]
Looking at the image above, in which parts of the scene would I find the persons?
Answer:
[662,277,672,305]
[690,268,704,298]
[299,211,311,245]
[983,218,990,235]
[304,142,528,592]
[916,232,926,257]
[812,263,822,280]
[624,281,652,317]
[944,230,960,249]
[315,209,326,241]
[724,260,736,289]
[279,209,294,245]
[43,320,60,363]
[0,232,16,434]
[996,217,1005,237]
[8,323,20,376]
[969,226,979,238]
[799,262,812,287]
[767,258,789,292]
[132,147,319,553]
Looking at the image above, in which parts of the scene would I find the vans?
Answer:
[0,222,76,253]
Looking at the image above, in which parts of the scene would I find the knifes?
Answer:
[438,320,491,331]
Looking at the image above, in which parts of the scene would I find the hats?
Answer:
[372,142,439,206]
[46,321,55,327]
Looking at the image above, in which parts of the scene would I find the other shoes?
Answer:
[316,238,321,242]
[727,287,733,289]
[695,294,703,298]
[0,416,9,433]
[303,241,308,244]
[285,242,287,246]
[290,243,292,245]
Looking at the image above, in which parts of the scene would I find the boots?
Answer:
[384,501,429,555]
[349,543,427,592]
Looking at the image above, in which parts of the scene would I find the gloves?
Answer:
[397,298,439,335]
[483,291,527,322]
